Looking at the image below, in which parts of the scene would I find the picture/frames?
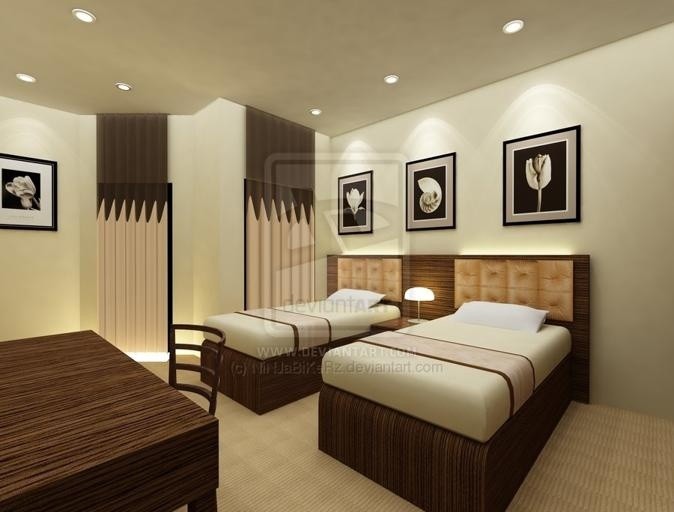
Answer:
[1,152,58,231]
[337,170,373,234]
[502,125,581,225]
[405,152,456,229]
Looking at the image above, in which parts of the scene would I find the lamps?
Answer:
[404,286,435,323]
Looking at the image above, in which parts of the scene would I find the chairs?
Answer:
[168,322,227,416]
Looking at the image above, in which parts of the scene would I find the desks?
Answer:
[1,330,219,511]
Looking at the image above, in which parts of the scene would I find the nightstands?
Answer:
[371,316,418,334]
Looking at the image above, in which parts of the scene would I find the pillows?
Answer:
[453,301,549,333]
[326,288,387,311]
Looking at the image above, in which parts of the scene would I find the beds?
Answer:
[318,312,574,512]
[200,300,401,415]
